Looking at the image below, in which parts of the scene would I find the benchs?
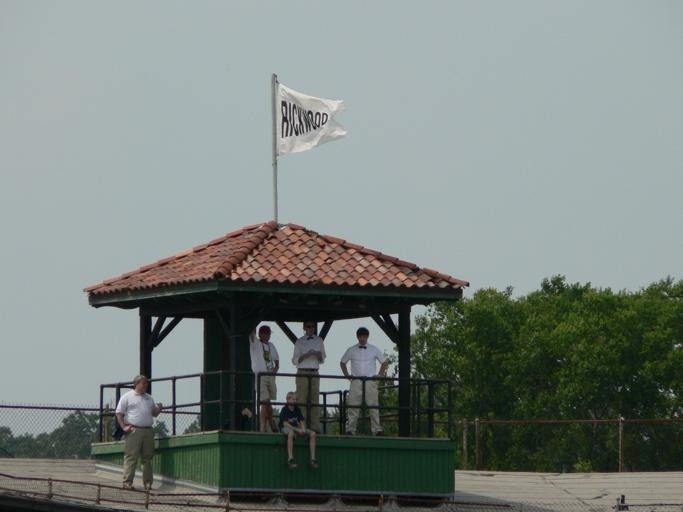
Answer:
[272,82,348,158]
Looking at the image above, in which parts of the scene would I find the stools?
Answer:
[297,368,319,372]
[131,425,153,430]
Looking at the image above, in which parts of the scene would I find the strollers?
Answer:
[358,345,368,350]
[306,336,315,341]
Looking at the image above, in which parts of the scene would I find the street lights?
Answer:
[110,415,125,442]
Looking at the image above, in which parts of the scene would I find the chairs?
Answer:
[142,482,153,492]
[288,457,298,469]
[371,428,385,437]
[344,430,356,435]
[122,480,135,490]
[310,457,321,469]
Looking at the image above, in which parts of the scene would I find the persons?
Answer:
[291,318,326,436]
[337,325,390,436]
[247,324,280,433]
[116,374,163,491]
[277,390,320,471]
